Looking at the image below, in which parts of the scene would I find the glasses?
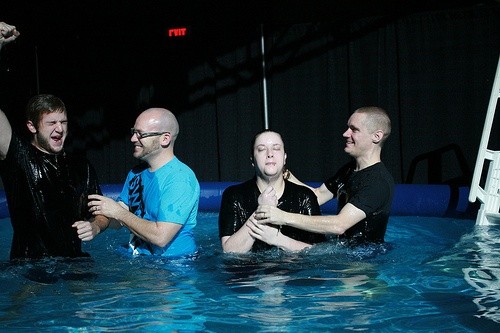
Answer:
[130,128,170,138]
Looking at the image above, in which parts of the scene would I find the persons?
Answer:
[255,108,394,247]
[218,130,327,256]
[86,109,200,258]
[0,20,109,262]
